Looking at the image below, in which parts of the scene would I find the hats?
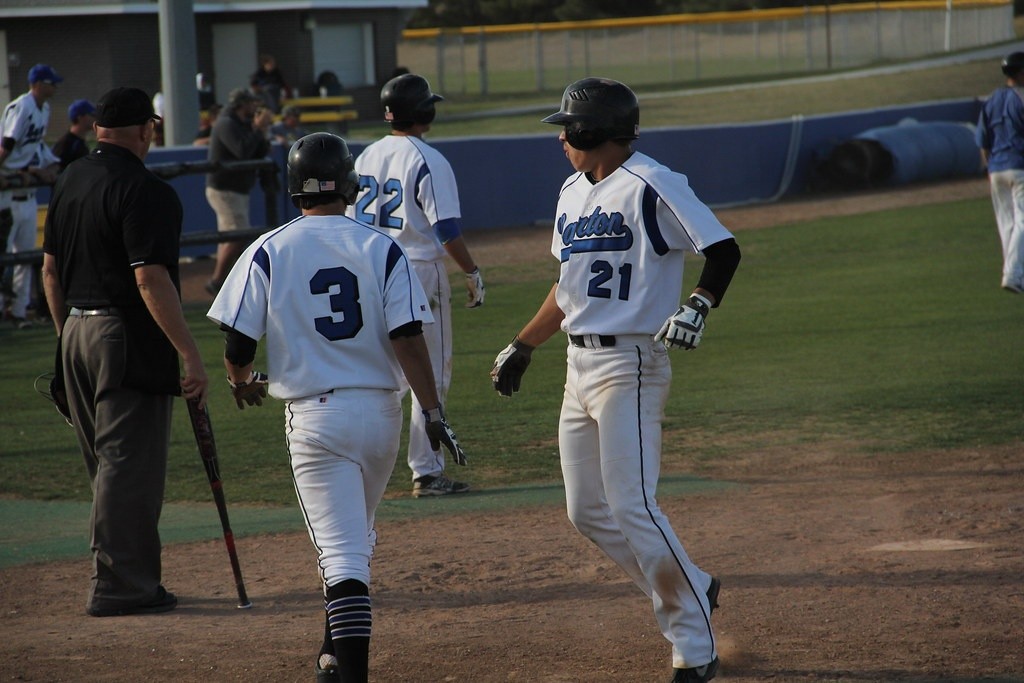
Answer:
[96,87,162,128]
[66,98,97,123]
[229,88,260,109]
[26,63,65,86]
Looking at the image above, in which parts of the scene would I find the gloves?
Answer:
[421,404,467,466]
[653,293,711,352]
[489,335,536,398]
[464,264,487,308]
[226,372,270,410]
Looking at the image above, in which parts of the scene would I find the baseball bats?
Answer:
[183,381,254,612]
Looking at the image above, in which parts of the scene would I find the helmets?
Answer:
[380,73,444,132]
[540,77,641,151]
[287,132,360,207]
[1002,52,1024,84]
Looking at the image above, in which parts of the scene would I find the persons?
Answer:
[0,64,97,329]
[43,87,208,616]
[345,72,484,499]
[492,78,743,683]
[206,87,272,301]
[975,50,1024,293]
[151,56,308,149]
[206,131,471,683]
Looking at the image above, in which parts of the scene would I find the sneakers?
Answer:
[412,474,472,499]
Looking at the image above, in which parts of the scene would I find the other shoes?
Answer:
[316,650,342,683]
[671,655,720,683]
[85,578,177,617]
[705,577,721,617]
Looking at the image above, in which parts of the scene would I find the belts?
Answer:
[569,334,616,348]
[69,306,125,318]
